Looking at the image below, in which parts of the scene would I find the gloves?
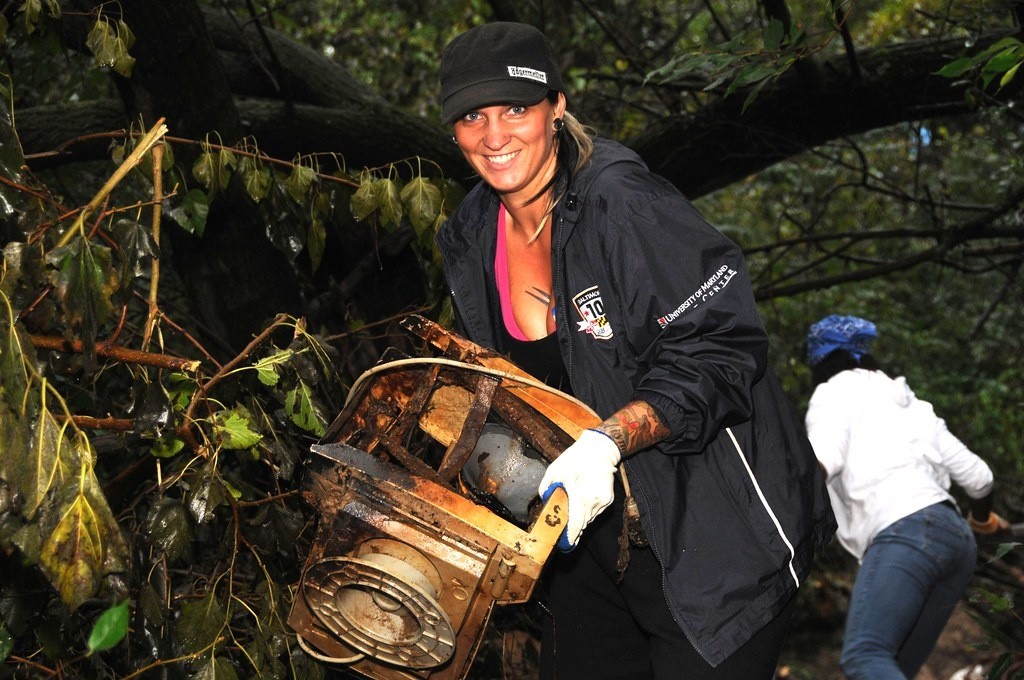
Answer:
[964,510,1011,535]
[538,426,623,554]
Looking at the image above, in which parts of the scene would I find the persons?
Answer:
[804,315,995,680]
[434,20,838,680]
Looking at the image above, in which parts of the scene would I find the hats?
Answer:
[438,21,566,126]
